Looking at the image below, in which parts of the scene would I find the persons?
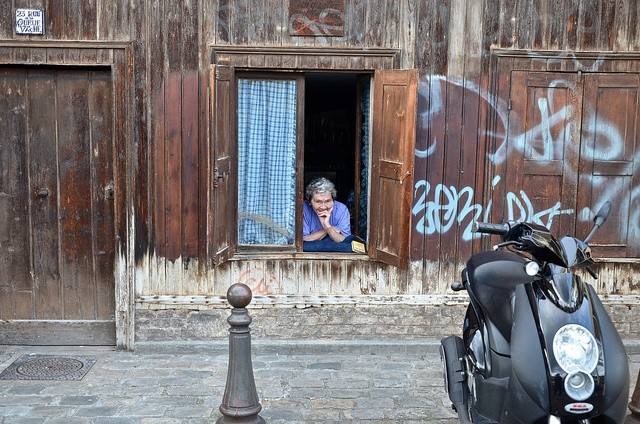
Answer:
[303,177,351,243]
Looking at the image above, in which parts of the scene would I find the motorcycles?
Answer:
[440,201,629,424]
